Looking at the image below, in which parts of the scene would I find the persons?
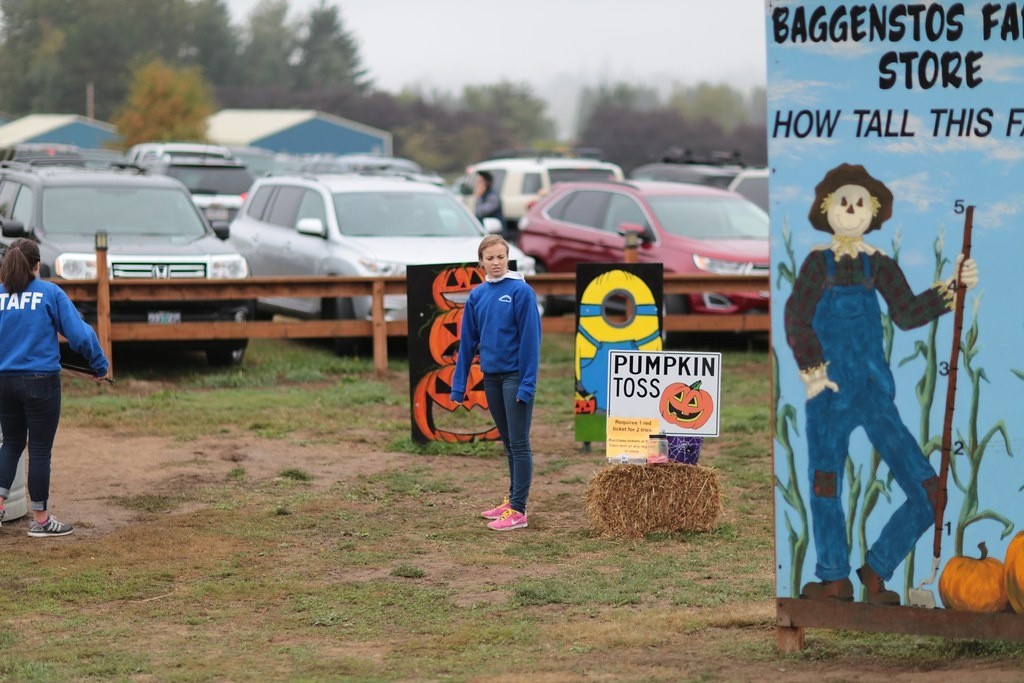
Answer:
[0,239,110,537]
[451,235,544,532]
[472,170,507,239]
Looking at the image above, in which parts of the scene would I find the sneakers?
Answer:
[0,506,6,527]
[26,514,75,537]
[481,503,511,519]
[486,509,528,532]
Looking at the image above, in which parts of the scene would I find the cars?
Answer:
[224,172,545,355]
[515,180,773,345]
[3,139,451,239]
[452,157,626,247]
[630,163,769,209]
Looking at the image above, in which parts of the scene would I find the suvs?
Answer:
[0,158,253,367]
